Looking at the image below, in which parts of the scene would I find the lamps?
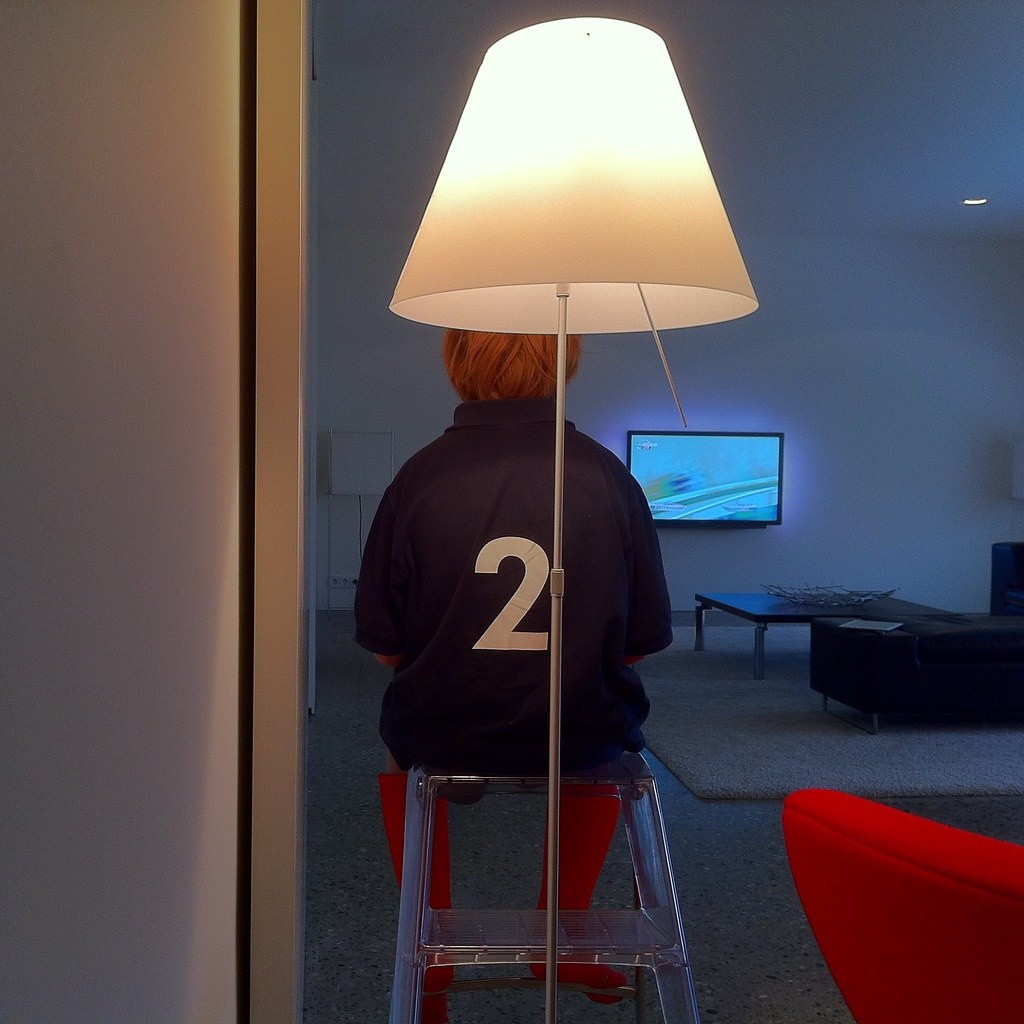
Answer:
[389,14,762,1024]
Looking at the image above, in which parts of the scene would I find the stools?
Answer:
[385,751,700,1024]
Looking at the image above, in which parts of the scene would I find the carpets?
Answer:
[630,624,1024,797]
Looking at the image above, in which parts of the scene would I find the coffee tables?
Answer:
[691,591,961,679]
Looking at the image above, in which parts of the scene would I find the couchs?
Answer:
[990,542,1024,616]
[811,616,1024,732]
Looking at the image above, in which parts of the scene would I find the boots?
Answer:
[532,781,630,1003]
[376,770,458,1024]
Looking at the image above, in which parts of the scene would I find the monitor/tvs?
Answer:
[626,430,784,528]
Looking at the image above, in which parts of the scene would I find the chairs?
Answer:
[783,787,1023,1024]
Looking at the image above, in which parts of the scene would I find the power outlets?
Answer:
[341,577,351,588]
[351,577,358,588]
[330,577,341,588]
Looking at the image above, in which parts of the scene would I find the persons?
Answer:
[352,328,674,1024]
